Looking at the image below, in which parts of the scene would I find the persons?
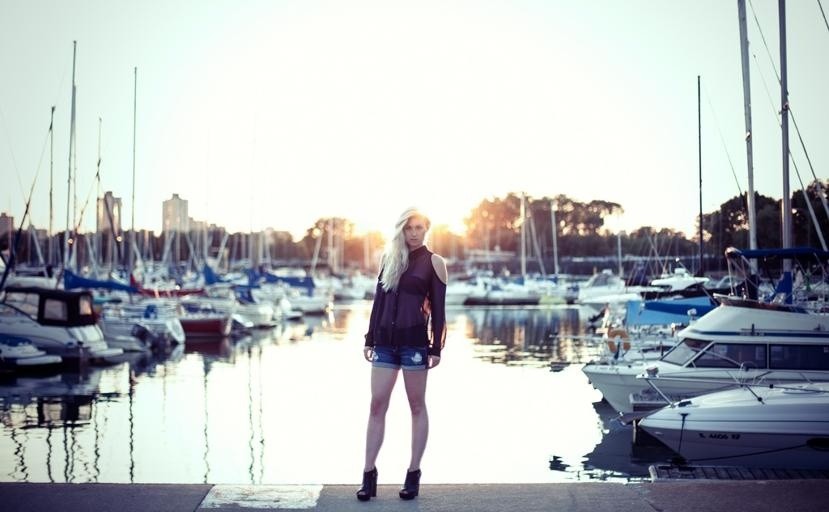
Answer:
[353,204,449,504]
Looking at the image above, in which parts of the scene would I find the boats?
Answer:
[569,246,828,423]
[636,369,829,465]
[448,191,828,324]
[558,427,649,484]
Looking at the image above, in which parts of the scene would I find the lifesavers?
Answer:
[608,327,631,353]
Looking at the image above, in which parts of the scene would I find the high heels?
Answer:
[399,468,421,500]
[357,467,378,501]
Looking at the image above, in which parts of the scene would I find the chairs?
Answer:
[725,345,757,369]
[706,345,740,365]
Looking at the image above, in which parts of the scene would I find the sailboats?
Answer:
[186,311,345,483]
[0,356,136,484]
[0,41,382,374]
[674,1,829,367]
